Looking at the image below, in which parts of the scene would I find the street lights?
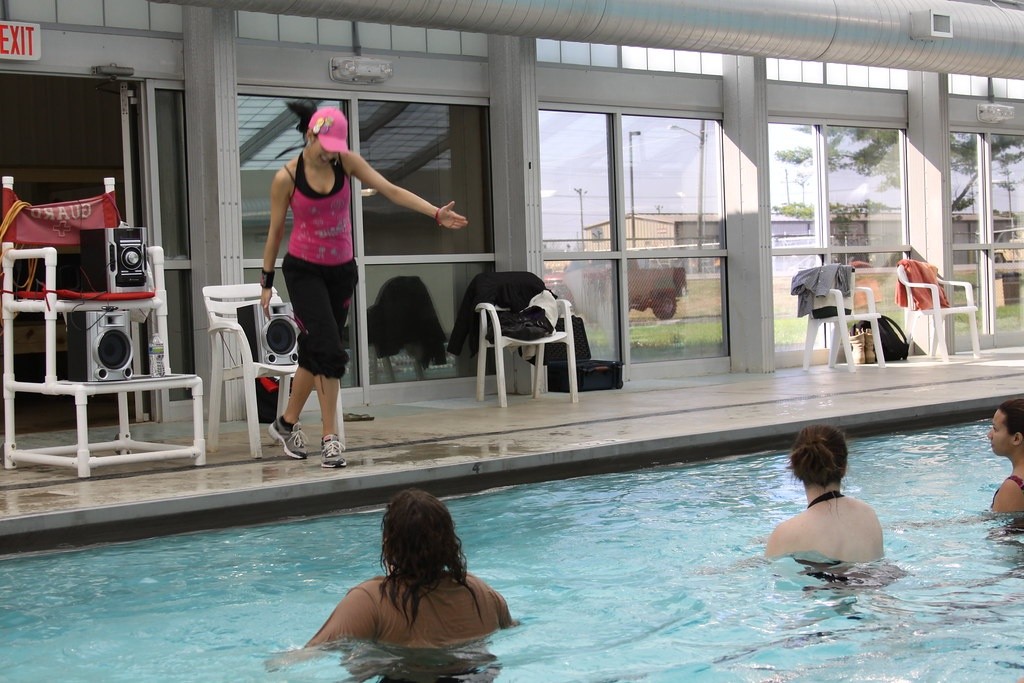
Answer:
[669,125,705,273]
[629,131,642,248]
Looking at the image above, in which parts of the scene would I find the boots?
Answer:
[848,329,876,364]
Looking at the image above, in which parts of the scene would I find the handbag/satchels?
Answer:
[254,375,281,423]
[521,289,559,333]
[485,305,554,344]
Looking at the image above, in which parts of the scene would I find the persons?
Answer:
[259,105,470,468]
[764,424,885,563]
[986,398,1024,513]
[304,488,522,647]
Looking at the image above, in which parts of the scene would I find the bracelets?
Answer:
[435,207,443,226]
[259,268,275,289]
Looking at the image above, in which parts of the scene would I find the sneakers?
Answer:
[319,433,347,468]
[267,416,310,459]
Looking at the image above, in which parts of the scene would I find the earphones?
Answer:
[306,141,311,148]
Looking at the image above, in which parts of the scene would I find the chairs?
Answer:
[802,267,885,372]
[899,264,979,360]
[202,282,345,460]
[472,271,579,407]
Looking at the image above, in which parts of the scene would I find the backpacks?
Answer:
[849,315,909,360]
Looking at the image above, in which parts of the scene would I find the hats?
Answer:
[308,106,349,153]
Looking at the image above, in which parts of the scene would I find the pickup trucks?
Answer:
[543,260,685,320]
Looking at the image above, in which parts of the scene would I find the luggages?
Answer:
[518,314,624,392]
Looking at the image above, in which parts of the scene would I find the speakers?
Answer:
[67,311,134,381]
[79,227,148,293]
[237,303,298,364]
